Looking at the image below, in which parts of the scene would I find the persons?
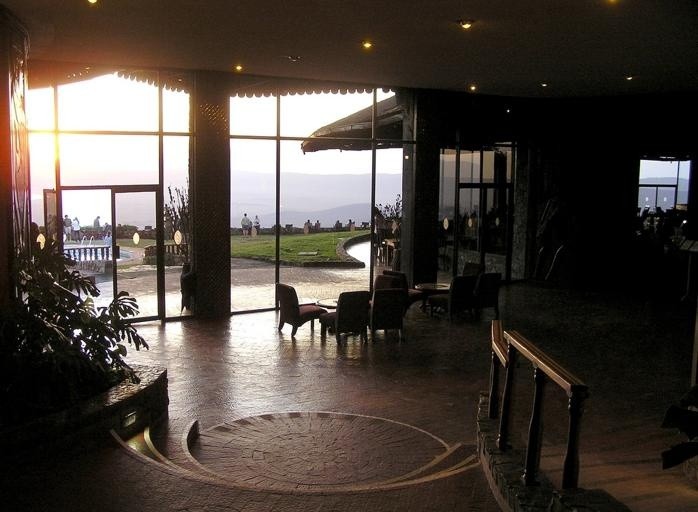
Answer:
[248,221,253,230]
[72,219,81,242]
[104,230,112,251]
[373,207,385,267]
[252,214,261,235]
[94,216,100,228]
[240,213,250,236]
[63,214,72,236]
[104,222,112,229]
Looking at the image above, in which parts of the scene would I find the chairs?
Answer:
[277,264,502,349]
